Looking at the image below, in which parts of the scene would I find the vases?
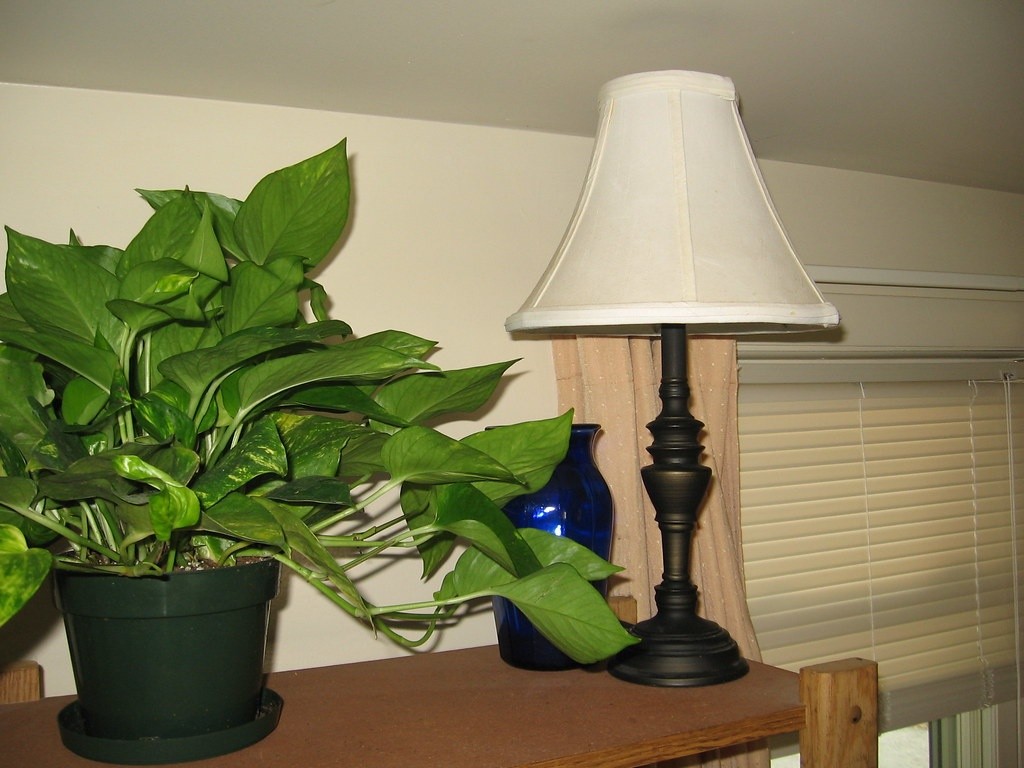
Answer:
[484,424,615,672]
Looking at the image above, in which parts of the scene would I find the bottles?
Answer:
[484,423,615,673]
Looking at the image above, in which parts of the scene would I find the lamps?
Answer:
[503,68,841,688]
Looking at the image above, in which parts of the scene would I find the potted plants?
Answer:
[0,137,643,768]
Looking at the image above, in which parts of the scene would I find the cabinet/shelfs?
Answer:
[0,595,880,768]
